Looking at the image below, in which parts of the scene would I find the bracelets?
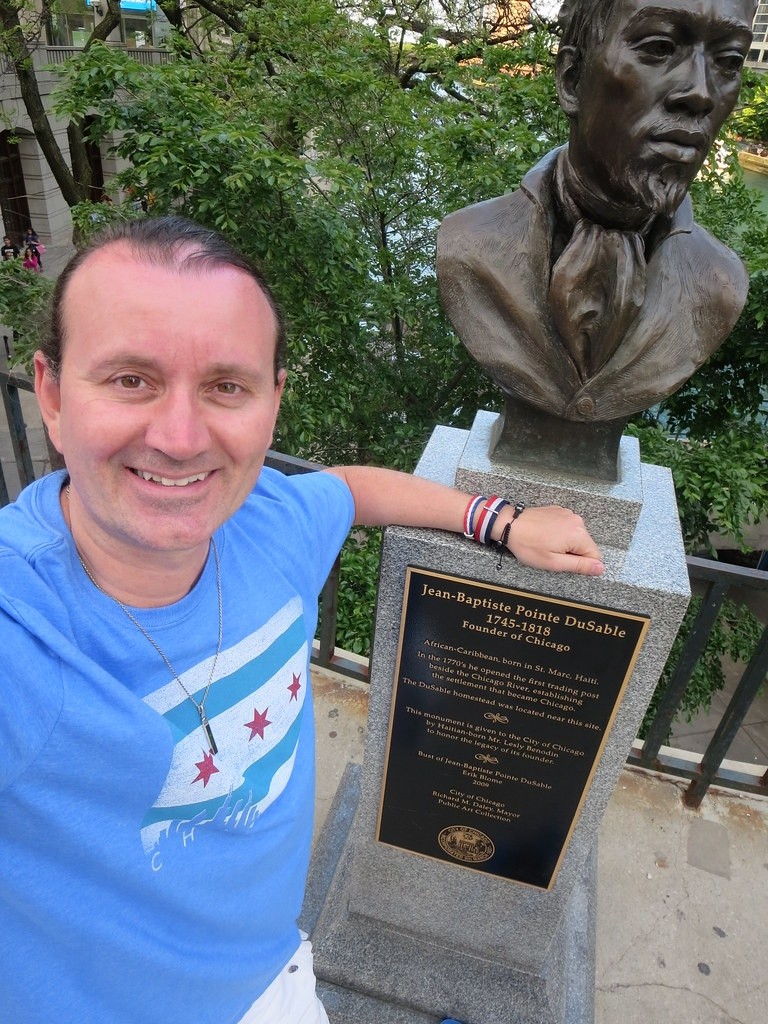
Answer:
[463,495,510,546]
[496,501,525,571]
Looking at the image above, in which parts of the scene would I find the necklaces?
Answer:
[66,481,223,755]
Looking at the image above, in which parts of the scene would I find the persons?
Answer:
[436,0,759,423]
[1,228,43,273]
[0,215,607,1024]
